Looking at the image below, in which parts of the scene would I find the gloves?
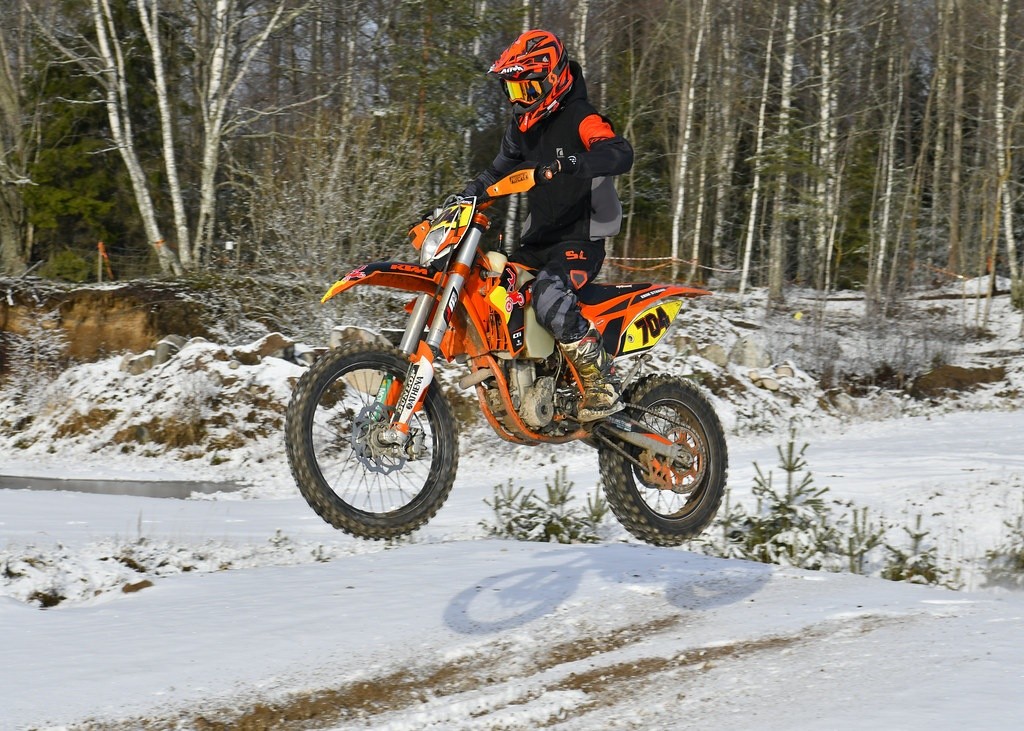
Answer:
[533,158,560,186]
[462,181,481,197]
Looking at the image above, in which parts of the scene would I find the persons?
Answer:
[421,29,635,423]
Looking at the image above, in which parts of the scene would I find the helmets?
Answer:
[487,28,574,133]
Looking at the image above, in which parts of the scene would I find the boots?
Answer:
[558,317,627,422]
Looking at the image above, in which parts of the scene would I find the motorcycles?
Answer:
[285,166,727,546]
